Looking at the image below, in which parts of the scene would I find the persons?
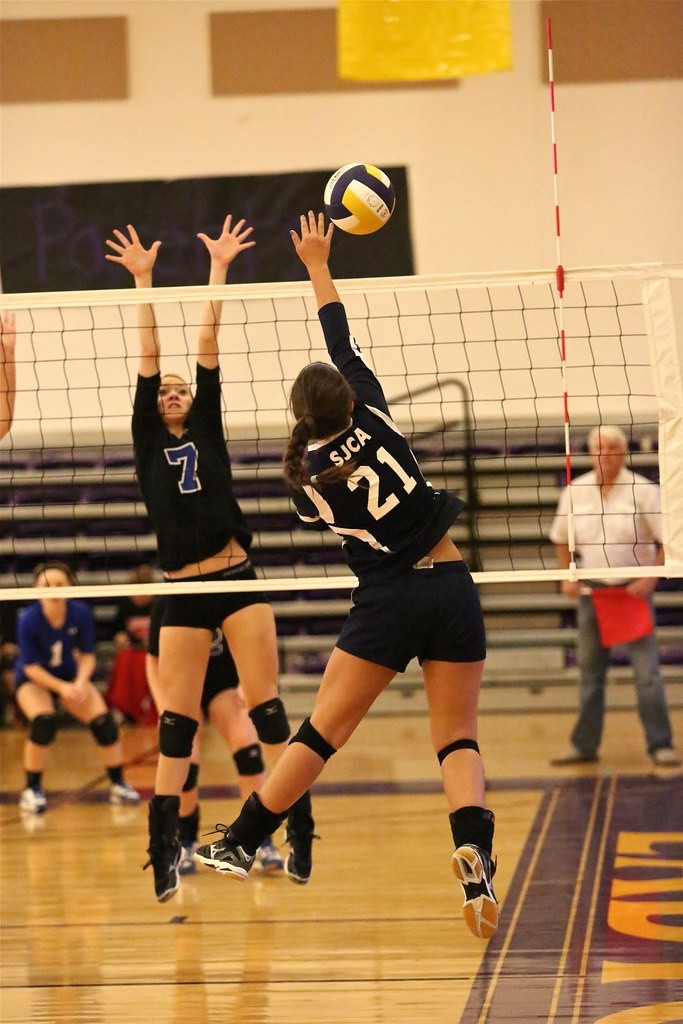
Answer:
[0,309,160,814]
[106,213,314,906]
[192,208,503,941]
[145,593,285,874]
[549,424,681,768]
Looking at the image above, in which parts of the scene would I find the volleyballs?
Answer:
[323,163,396,235]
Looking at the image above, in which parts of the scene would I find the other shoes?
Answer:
[19,788,48,813]
[651,745,678,766]
[550,744,598,764]
[110,785,141,807]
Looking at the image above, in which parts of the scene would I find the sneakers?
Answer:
[142,837,187,902]
[284,826,321,887]
[251,844,285,870]
[195,823,255,880]
[452,842,500,939]
[177,840,200,874]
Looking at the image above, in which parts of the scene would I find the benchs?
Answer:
[0,433,683,690]
